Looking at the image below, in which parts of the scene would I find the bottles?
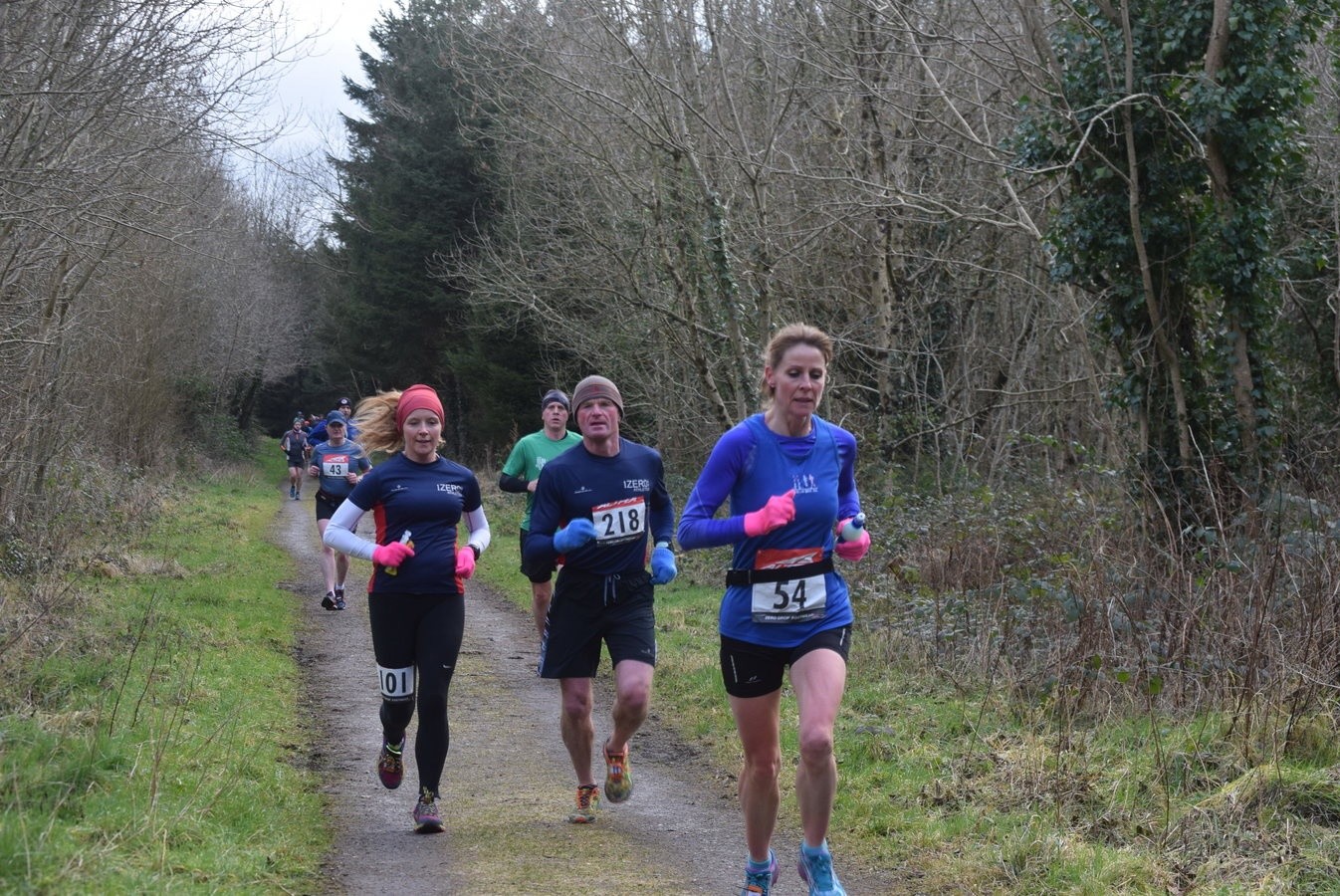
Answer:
[832,512,866,551]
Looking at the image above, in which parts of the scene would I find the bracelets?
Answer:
[655,541,674,552]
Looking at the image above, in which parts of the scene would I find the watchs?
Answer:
[468,543,480,561]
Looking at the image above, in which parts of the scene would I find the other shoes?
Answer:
[295,494,300,500]
[290,487,295,498]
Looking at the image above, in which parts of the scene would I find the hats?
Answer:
[305,421,310,427]
[326,410,347,426]
[572,375,624,425]
[395,384,445,434]
[293,418,302,424]
[338,398,353,407]
[542,389,570,412]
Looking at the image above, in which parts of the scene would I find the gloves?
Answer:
[836,518,872,562]
[552,518,597,552]
[456,546,475,579]
[650,548,677,584]
[744,489,796,538]
[373,542,415,566]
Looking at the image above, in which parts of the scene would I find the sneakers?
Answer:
[378,731,406,789]
[322,591,338,611]
[335,586,346,610]
[413,787,447,834]
[741,849,779,896]
[797,844,846,896]
[603,736,633,803]
[568,782,601,824]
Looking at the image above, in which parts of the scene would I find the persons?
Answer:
[307,410,373,610]
[500,390,588,673]
[677,324,869,896]
[296,398,361,480]
[525,376,675,823]
[280,418,311,500]
[324,385,490,833]
